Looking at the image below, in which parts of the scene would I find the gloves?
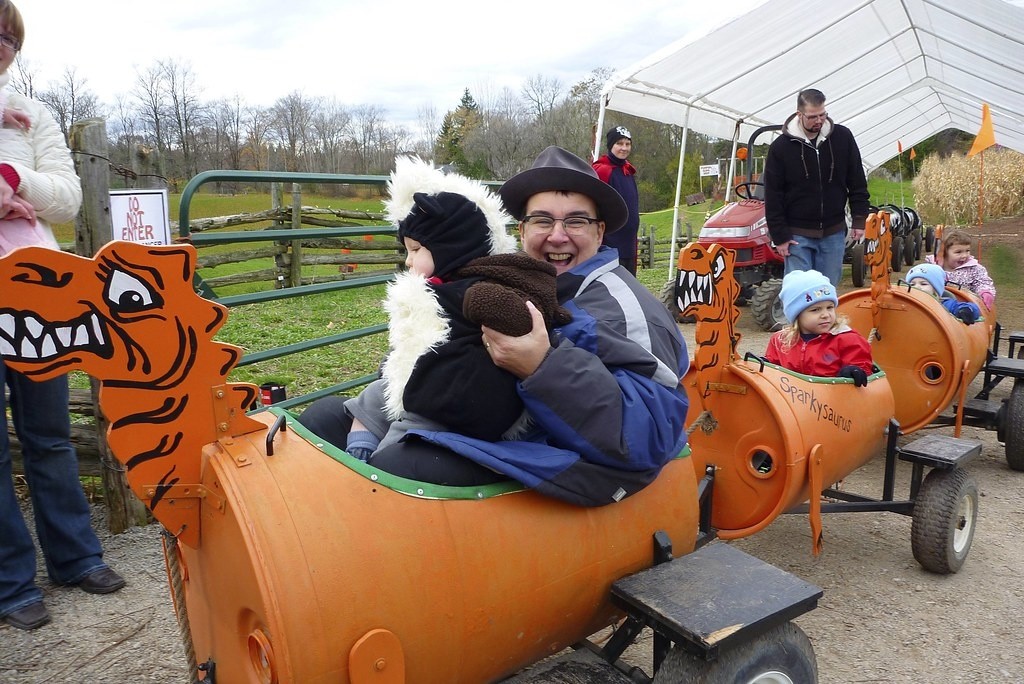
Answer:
[958,307,975,325]
[836,366,867,387]
[979,291,995,312]
[758,357,769,363]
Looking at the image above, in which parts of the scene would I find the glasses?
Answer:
[522,214,600,236]
[803,112,828,121]
[0,33,21,51]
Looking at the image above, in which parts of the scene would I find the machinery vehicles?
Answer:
[658,125,935,332]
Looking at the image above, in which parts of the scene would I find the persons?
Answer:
[906,263,980,379]
[758,269,873,387]
[764,89,870,287]
[924,231,997,312]
[296,145,690,508]
[347,152,557,462]
[0,0,128,634]
[590,126,639,277]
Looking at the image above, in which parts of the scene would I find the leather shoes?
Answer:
[4,601,52,630]
[80,568,126,593]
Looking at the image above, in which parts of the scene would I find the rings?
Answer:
[484,343,489,348]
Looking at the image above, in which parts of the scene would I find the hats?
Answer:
[496,145,629,236]
[778,269,838,323]
[607,126,632,151]
[906,263,946,297]
